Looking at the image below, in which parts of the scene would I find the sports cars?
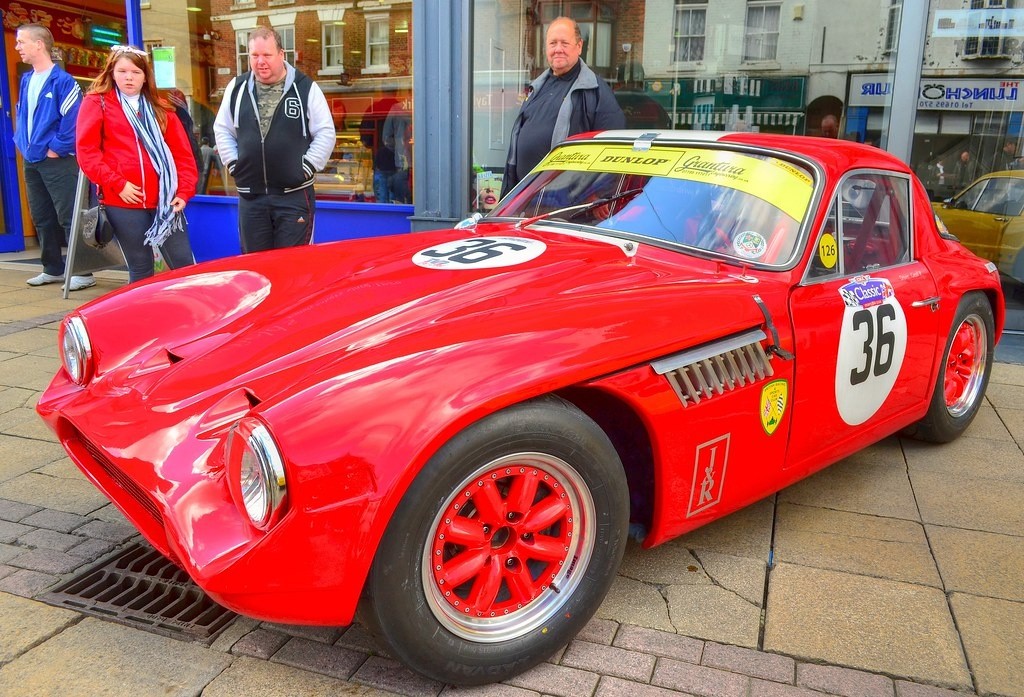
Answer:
[35,129,1005,690]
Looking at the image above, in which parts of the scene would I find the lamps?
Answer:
[203,28,222,40]
[334,58,362,87]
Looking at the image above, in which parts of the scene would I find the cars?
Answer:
[929,169,1024,291]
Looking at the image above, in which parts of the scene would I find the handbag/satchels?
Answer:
[82,206,114,250]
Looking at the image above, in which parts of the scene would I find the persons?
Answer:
[213,26,338,256]
[75,43,201,284]
[331,77,410,203]
[11,24,96,291]
[497,15,627,222]
[821,114,838,139]
[613,60,673,130]
[933,136,1024,188]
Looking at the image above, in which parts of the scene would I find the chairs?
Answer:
[597,178,715,250]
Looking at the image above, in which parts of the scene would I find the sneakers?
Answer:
[27,272,66,286]
[61,273,96,291]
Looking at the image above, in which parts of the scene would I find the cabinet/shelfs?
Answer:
[211,136,373,191]
